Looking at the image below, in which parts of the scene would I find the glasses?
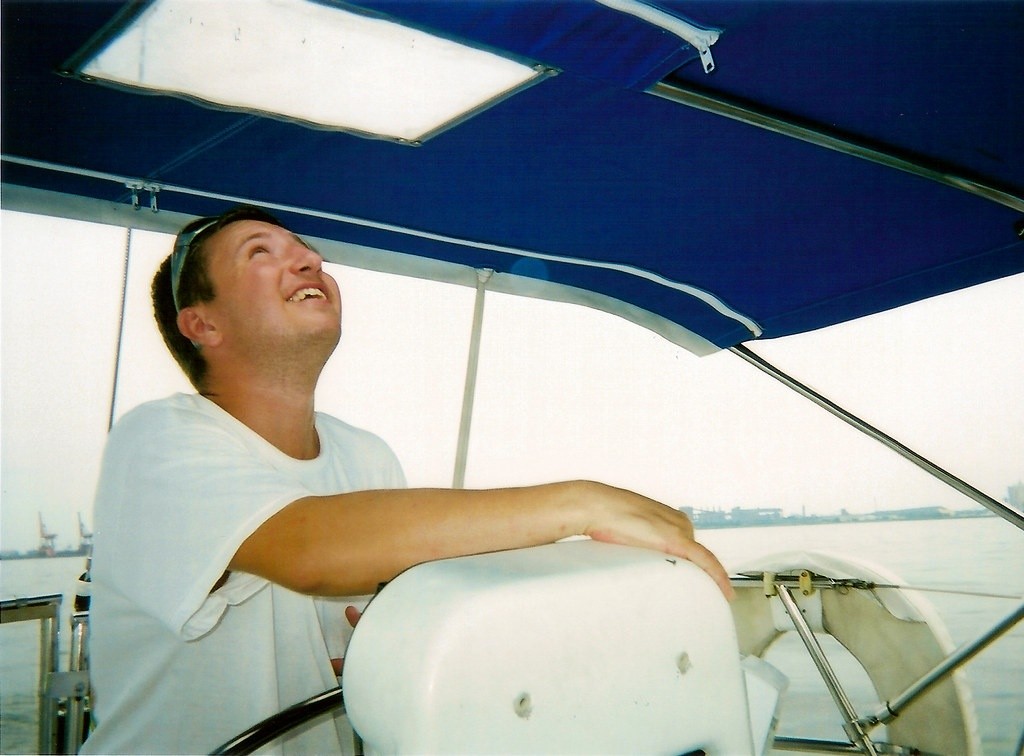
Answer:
[171,217,220,349]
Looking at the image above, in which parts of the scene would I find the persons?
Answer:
[74,214,736,756]
